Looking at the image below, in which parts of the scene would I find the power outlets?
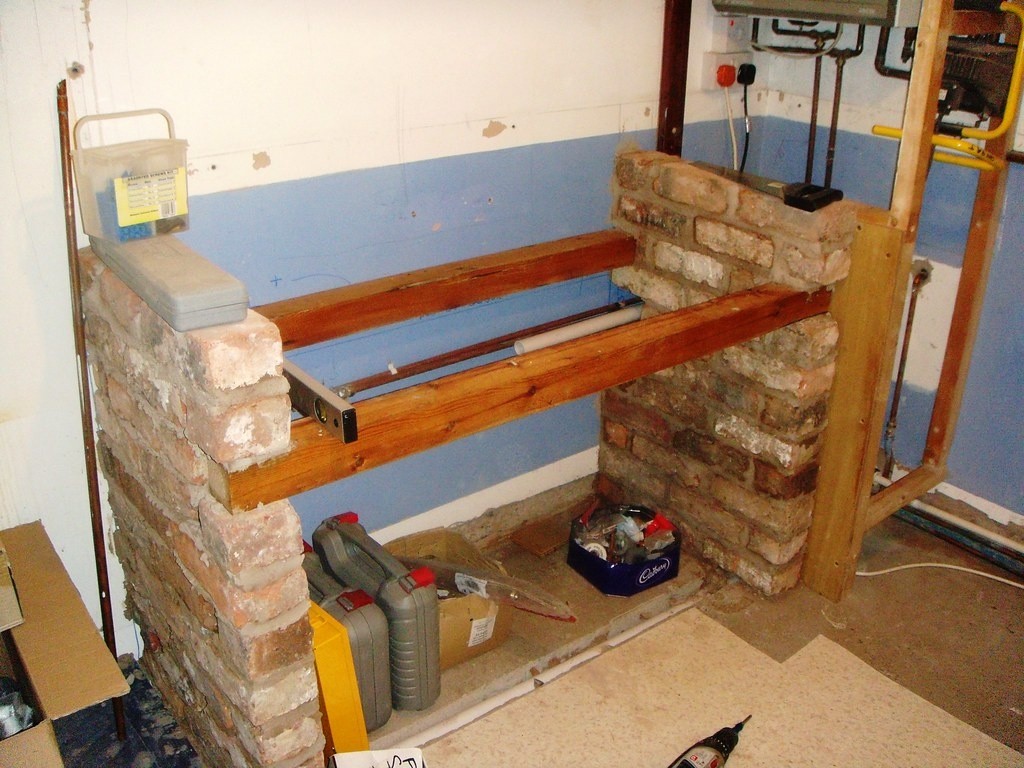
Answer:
[701,51,753,91]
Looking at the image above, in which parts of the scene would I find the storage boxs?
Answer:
[568,505,682,597]
[328,748,428,768]
[381,527,514,674]
[0,520,132,768]
[313,511,442,713]
[303,539,393,733]
[309,599,370,768]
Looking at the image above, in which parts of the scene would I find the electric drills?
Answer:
[668,713,753,768]
